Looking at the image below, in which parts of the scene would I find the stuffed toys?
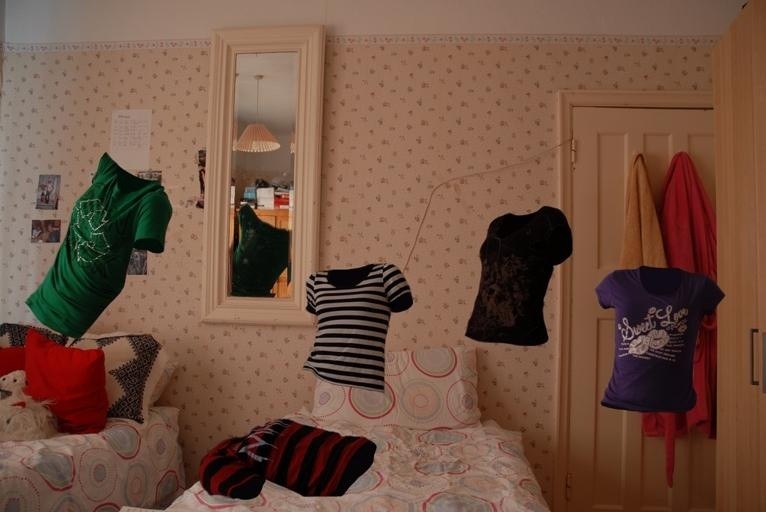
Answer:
[0,371,57,441]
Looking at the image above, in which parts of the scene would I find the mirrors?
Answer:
[197,22,328,330]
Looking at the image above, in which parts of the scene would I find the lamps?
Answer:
[236,76,280,154]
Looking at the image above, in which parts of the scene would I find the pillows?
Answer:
[66,331,163,431]
[311,345,484,429]
[0,322,66,352]
[22,328,109,436]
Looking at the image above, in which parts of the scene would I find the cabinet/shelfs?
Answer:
[234,204,291,297]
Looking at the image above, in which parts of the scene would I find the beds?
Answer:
[1,320,184,510]
[160,409,551,512]
